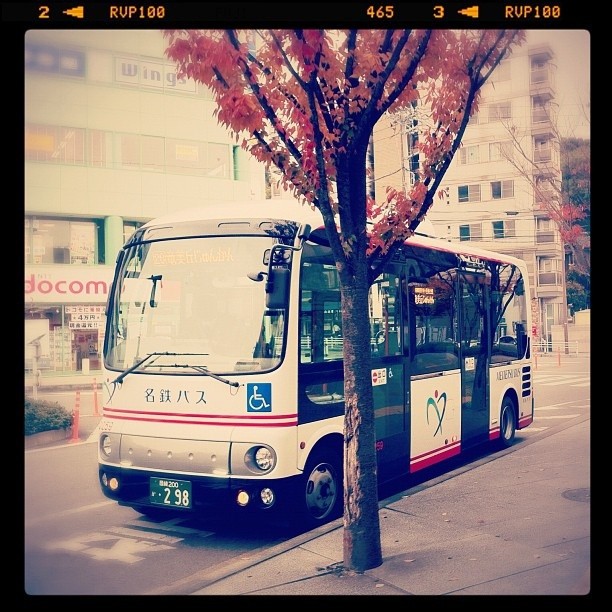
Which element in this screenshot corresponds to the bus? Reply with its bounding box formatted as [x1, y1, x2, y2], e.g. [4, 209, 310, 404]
[97, 199, 533, 529]
